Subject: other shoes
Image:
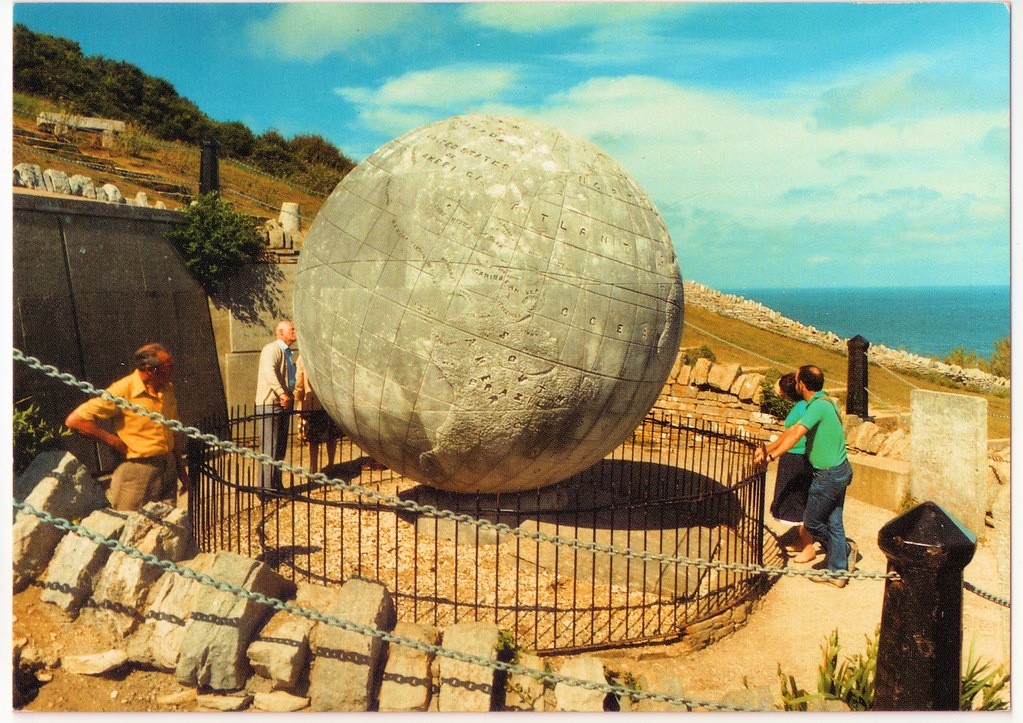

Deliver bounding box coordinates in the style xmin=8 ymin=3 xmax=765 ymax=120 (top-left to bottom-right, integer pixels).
xmin=271 ymin=482 xmax=286 ymax=490
xmin=847 ymin=541 xmax=858 ymax=574
xmin=307 ymin=477 xmax=319 ymax=489
xmin=785 ymin=539 xmax=804 ymax=552
xmin=809 ymin=574 xmax=849 ymax=589
xmin=327 ymin=465 xmax=339 ymax=476
xmin=256 ymin=493 xmax=271 ymax=502
xmin=793 ymin=548 xmax=816 ymax=563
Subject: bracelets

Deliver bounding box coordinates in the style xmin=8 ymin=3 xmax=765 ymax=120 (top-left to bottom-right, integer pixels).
xmin=762 ymin=446 xmax=768 ymax=454
xmin=769 ymin=454 xmax=774 ymax=460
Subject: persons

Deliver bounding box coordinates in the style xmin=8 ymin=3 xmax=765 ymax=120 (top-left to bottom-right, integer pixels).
xmin=256 ymin=321 xmax=297 ymax=498
xmin=294 ymin=354 xmax=338 ymax=474
xmin=754 ymin=366 xmax=857 ymax=586
xmin=64 ymin=343 xmax=191 ymax=511
